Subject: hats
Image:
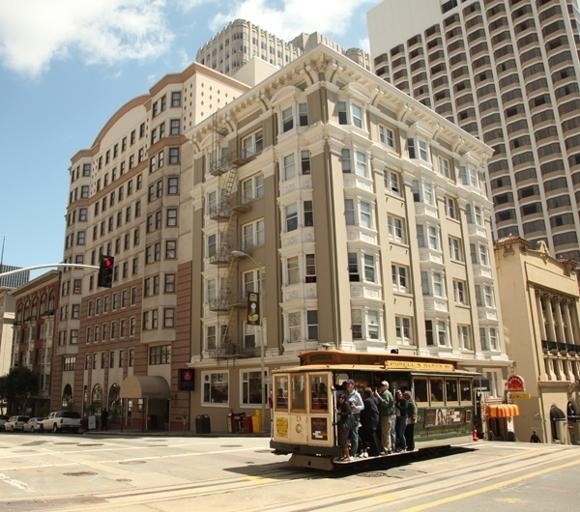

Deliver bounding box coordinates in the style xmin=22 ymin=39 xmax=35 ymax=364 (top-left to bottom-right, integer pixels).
xmin=344 ymin=379 xmax=354 ymax=384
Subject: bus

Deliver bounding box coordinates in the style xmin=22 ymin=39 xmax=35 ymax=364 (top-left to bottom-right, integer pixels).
xmin=269 ymin=348 xmax=481 ymax=470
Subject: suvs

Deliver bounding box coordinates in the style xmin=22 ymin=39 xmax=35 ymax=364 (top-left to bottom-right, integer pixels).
xmin=41 ymin=410 xmax=83 ymax=434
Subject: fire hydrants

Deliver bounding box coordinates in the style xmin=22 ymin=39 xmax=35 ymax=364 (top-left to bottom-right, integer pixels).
xmin=472 ymin=426 xmax=479 ymax=440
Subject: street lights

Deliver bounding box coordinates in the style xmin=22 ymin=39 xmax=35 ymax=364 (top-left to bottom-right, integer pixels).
xmin=229 ymin=249 xmax=267 ymax=434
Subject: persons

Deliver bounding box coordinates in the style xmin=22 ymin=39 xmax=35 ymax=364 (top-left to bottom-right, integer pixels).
xmin=101 ymin=407 xmax=109 ymax=431
xmin=528 ymin=430 xmax=542 ymax=443
xmin=276 ymin=378 xmax=417 ymax=462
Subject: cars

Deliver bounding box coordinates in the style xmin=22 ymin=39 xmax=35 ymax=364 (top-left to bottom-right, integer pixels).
xmin=0 ymin=414 xmax=44 ymax=432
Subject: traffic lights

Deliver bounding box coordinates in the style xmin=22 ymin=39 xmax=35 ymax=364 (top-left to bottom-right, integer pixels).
xmin=96 ymin=254 xmax=115 ymax=288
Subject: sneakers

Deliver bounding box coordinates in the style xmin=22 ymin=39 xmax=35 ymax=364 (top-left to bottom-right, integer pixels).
xmin=340 ymin=448 xmax=415 ymax=462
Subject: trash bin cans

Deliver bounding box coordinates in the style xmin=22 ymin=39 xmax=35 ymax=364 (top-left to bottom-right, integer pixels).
xmin=196 ymin=415 xmax=210 ymax=433
